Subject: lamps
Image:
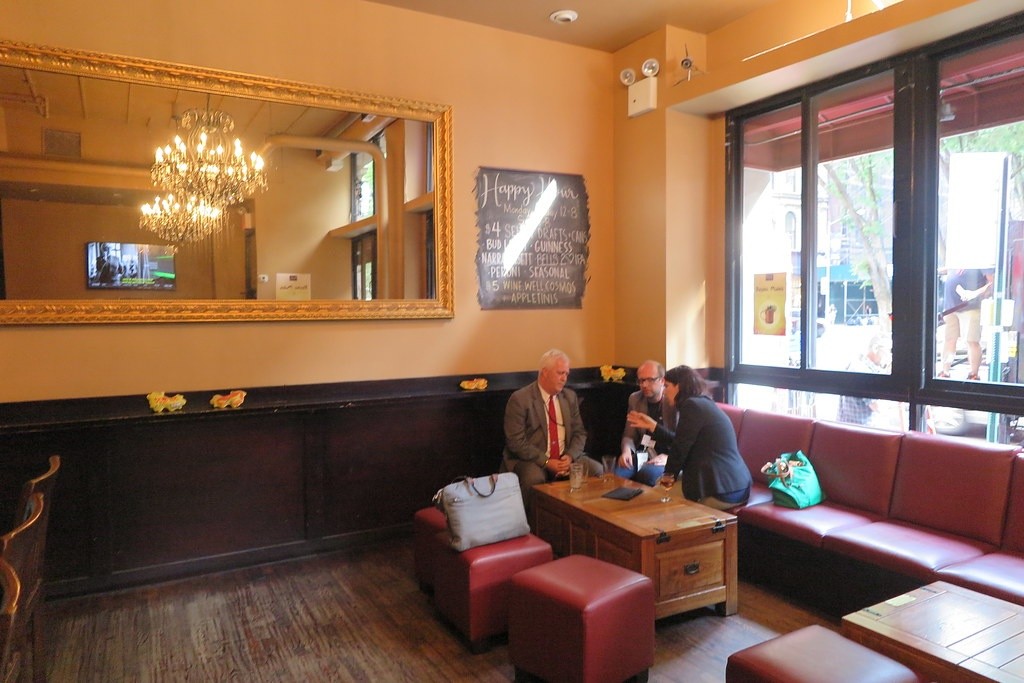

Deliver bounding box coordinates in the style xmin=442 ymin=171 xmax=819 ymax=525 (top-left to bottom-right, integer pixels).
xmin=136 ymin=93 xmax=270 ymax=244
xmin=619 ymin=58 xmax=660 ymax=117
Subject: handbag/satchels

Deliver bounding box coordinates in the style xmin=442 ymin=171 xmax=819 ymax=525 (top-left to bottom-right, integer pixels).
xmin=432 ymin=472 xmax=530 ymax=552
xmin=760 ymin=449 xmax=823 ymax=509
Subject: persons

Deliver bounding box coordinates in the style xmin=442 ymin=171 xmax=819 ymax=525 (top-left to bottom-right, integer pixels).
xmin=499 ymin=349 xmax=604 ymax=511
xmin=627 ymin=365 xmax=753 ymax=511
xmin=613 ymin=359 xmax=680 ymax=487
xmin=95 ymin=243 xmax=137 ymax=283
xmin=938 ymin=215 xmax=994 ymax=381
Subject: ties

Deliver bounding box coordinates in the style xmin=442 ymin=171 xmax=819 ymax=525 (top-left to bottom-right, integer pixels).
xmin=548 ymin=396 xmax=561 ymax=460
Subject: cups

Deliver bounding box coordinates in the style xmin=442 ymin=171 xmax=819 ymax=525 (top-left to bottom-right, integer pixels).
xmin=568 ymin=458 xmax=589 ymax=492
xmin=602 ymin=455 xmax=616 ymax=482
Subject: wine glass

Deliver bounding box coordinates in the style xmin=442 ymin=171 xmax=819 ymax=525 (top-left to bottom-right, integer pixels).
xmin=661 ymin=472 xmax=675 ymax=502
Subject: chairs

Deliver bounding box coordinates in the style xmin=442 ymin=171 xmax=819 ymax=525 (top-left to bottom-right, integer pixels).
xmin=0 ymin=455 xmax=60 ymax=683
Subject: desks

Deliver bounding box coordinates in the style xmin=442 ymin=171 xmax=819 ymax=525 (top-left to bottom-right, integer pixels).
xmin=532 ymin=468 xmax=738 ymax=619
xmin=841 ymin=581 xmax=1024 ymax=683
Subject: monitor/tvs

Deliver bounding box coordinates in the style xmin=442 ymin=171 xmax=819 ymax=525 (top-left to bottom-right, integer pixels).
xmin=87 ymin=242 xmax=176 ymax=292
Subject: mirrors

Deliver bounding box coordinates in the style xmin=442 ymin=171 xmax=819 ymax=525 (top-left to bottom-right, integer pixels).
xmin=0 ymin=37 xmax=457 ymax=323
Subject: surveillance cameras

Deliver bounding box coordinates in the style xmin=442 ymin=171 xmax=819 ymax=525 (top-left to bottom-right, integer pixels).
xmin=681 ymin=57 xmax=693 ymax=69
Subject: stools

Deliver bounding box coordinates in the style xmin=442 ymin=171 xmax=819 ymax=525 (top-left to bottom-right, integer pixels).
xmin=507 ymin=551 xmax=657 ymax=682
xmin=413 ymin=506 xmax=455 ymax=591
xmin=726 ymin=623 xmax=918 ymax=683
xmin=430 ymin=530 xmax=552 ymax=644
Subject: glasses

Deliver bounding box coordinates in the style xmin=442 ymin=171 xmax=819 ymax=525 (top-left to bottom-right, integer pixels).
xmin=635 ymin=376 xmax=661 ymax=386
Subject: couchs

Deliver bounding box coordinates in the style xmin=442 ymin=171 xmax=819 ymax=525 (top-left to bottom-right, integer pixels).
xmin=714 ymin=400 xmax=1023 ymax=603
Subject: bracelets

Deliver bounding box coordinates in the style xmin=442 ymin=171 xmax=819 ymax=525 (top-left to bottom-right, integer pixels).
xmin=543 ymin=458 xmax=549 ymax=469
